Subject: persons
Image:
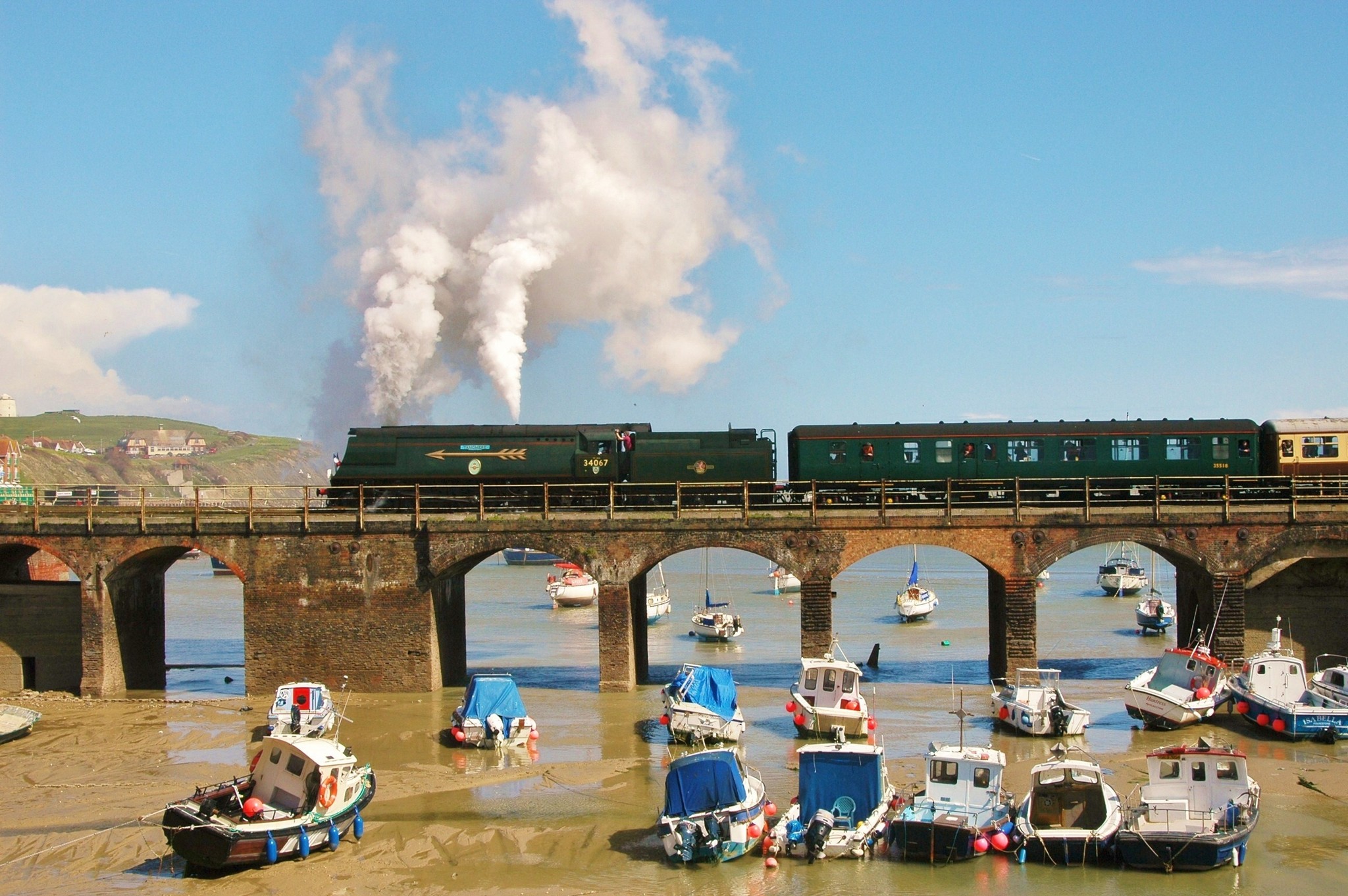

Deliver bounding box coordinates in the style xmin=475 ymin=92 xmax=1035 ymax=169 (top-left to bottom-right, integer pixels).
xmin=1238 ymin=438 xmax=1251 ymax=457
xmin=614 ymin=429 xmax=633 ymax=451
xmin=1302 ymin=438 xmax=1338 ymax=458
xmin=1282 ymin=439 xmax=1293 ymax=458
xmin=961 ymin=441 xmax=1083 ymax=461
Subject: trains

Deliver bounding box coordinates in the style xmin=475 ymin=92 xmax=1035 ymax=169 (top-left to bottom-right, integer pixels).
xmin=317 ymin=417 xmax=1348 ymax=503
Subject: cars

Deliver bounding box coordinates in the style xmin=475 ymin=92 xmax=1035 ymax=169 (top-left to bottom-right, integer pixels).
xmin=132 ymin=454 xmax=149 ymax=459
xmin=154 ymin=448 xmax=217 ymax=458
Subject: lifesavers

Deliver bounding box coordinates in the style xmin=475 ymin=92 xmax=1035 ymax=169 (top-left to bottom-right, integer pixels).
xmin=317 ymin=775 xmax=338 ymax=808
xmin=1191 ymin=676 xmax=1204 ymax=691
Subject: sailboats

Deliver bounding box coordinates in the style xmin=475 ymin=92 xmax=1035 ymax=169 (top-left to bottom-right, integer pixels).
xmin=687 ymin=548 xmax=745 ymax=640
xmin=890 ymin=544 xmax=940 ymax=622
xmin=1035 ymin=538 xmax=1176 ymax=634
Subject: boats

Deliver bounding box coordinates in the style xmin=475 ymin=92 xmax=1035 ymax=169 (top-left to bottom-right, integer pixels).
xmin=1220 ymin=614 xmax=1348 ymax=743
xmin=266 ymin=681 xmax=354 ymax=742
xmin=758 ymin=687 xmax=905 ymax=871
xmin=180 ymin=548 xmax=237 ymax=576
xmin=1004 ymin=742 xmax=1125 ymax=869
xmin=767 ymin=560 xmax=801 ymax=596
xmin=0 ymin=705 xmax=42 ymax=745
xmin=645 ymin=562 xmax=670 ymax=624
xmin=655 ymin=726 xmax=777 ymax=865
xmin=989 ymin=667 xmax=1094 ymax=738
xmin=502 ymin=547 xmax=569 ymax=566
xmin=157 ymin=730 xmax=377 ymax=874
xmin=785 ymin=631 xmax=872 ymax=740
xmin=658 ymin=662 xmax=746 ymax=745
xmin=885 ymin=687 xmax=1020 ymax=864
xmin=448 ymin=668 xmax=538 ymax=761
xmin=1106 ymin=734 xmax=1261 ymax=876
xmin=1116 ymin=576 xmax=1234 ymax=732
xmin=544 ymin=562 xmax=599 ymax=609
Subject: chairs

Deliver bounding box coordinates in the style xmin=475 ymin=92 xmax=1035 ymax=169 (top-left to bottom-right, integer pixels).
xmin=830 ymin=796 xmax=856 ymax=831
